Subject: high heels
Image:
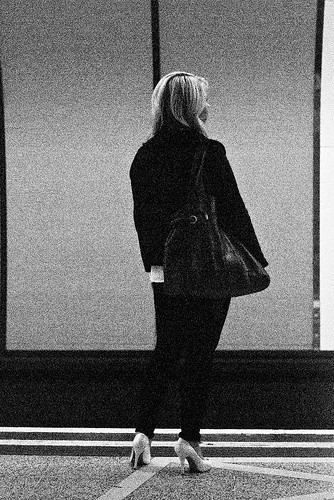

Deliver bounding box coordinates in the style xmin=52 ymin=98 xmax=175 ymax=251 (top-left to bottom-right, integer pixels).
xmin=129 ymin=433 xmax=153 ymax=469
xmin=172 ymin=438 xmax=213 ymax=474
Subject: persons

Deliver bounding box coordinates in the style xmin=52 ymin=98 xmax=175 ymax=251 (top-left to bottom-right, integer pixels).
xmin=125 ymin=71 xmax=271 ymax=471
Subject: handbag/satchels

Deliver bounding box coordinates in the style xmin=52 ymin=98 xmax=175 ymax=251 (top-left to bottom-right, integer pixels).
xmin=165 ymin=200 xmax=273 ymax=297
